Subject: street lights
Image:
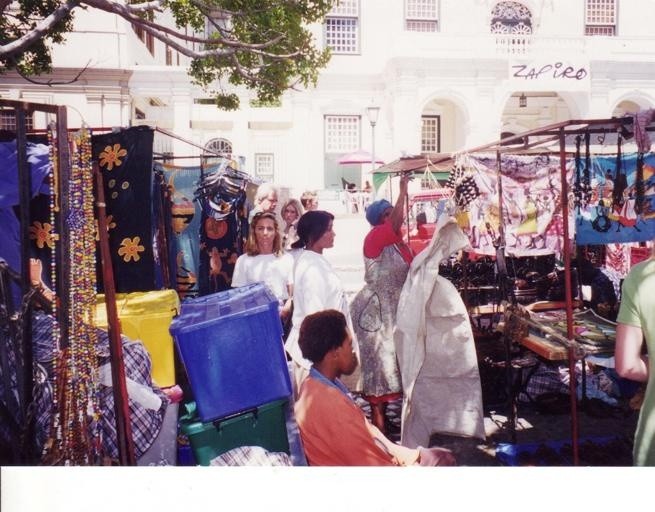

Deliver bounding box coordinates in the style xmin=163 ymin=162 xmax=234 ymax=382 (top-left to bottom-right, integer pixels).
xmin=366 ymin=106 xmax=382 ymax=173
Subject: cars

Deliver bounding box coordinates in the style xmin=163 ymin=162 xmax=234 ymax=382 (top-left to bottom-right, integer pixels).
xmin=398 ymin=187 xmax=455 ymax=259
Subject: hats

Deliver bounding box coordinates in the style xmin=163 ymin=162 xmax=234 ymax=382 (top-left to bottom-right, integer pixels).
xmin=365 ymin=199 xmax=392 ymax=227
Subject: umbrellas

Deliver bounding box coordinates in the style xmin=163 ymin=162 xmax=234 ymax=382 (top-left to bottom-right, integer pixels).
xmin=337 ymin=150 xmax=386 ymax=166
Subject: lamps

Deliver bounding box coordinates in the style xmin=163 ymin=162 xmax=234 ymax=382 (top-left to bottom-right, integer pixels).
xmin=520 ymin=93 xmax=527 ymax=107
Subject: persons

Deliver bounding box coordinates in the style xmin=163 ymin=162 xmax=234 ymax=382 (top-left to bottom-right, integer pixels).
xmin=346 ymin=171 xmax=417 ymax=434
xmin=362 ymin=180 xmax=373 ymax=213
xmin=230 ymin=210 xmax=294 ymax=359
xmin=245 ymin=182 xmax=283 ymax=246
xmin=279 ymin=197 xmax=305 ymax=251
xmin=300 ymin=190 xmax=319 ymax=214
xmin=282 ymin=209 xmax=362 ymax=396
xmin=348 ymin=182 xmax=360 ymax=213
xmin=291 ymin=308 xmax=460 ymax=466
xmin=613 ymin=239 xmax=655 ymax=466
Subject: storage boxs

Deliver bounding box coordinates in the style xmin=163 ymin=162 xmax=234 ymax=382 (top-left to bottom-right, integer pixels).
xmin=96 ymin=281 xmax=292 ymax=466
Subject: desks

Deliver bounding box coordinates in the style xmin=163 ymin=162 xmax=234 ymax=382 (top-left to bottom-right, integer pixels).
xmin=497 ymin=322 xmax=615 ymax=445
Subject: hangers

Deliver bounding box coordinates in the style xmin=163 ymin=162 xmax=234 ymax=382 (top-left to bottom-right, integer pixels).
xmin=192 ymin=156 xmax=254 ymax=203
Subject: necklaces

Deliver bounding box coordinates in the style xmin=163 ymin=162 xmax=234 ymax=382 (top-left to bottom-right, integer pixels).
xmin=43 ymin=120 xmax=106 ymax=466
xmin=573 ymin=131 xmax=646 ymax=217
